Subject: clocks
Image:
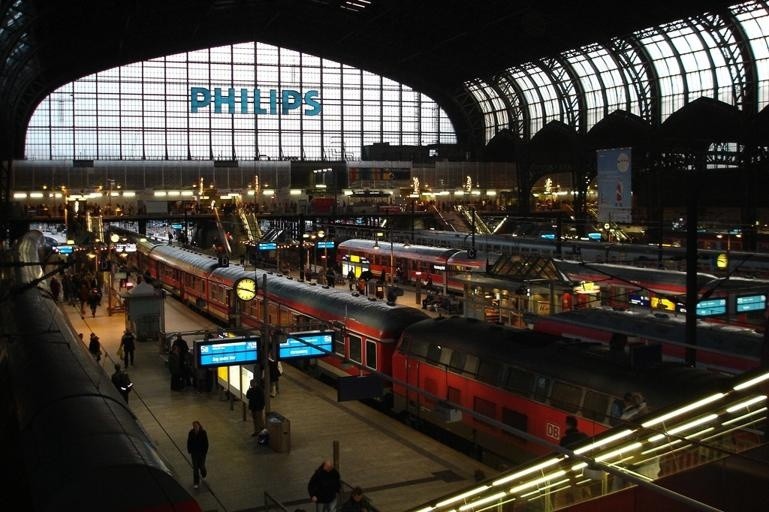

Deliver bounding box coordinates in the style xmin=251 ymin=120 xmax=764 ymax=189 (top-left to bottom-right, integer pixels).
xmin=233 ymin=278 xmax=257 ymax=301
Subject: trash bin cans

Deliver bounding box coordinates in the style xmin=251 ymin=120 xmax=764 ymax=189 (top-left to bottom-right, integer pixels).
xmin=265 ymin=412 xmax=290 ymax=453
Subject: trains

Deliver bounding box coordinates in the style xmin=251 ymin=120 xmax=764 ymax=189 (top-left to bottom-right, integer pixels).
xmin=103 ymin=222 xmax=769 ymax=511
xmin=0 ymin=230 xmax=203 ymax=512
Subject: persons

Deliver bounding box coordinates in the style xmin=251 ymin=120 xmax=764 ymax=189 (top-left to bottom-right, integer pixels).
xmin=89 ymin=332 xmax=102 ymax=364
xmin=49 ymin=216 xmax=124 ymax=319
xmin=620 ymin=391 xmax=666 ymax=486
xmin=608 ymin=389 xmax=630 ymax=491
xmin=537 ymin=198 xmax=574 ymax=211
xmin=143 ymin=199 xmax=385 ymax=295
xmin=169 ymin=333 xmax=193 ymax=390
xmin=245 ymin=376 xmax=265 ymax=438
xmin=339 ymin=487 xmax=378 ymax=512
xmin=307 ymin=461 xmax=340 ymax=512
xmin=117 ymin=328 xmax=137 ymax=368
xmin=186 ymin=420 xmax=210 ymax=491
xmin=63 ymin=199 xmax=125 ymax=217
xmin=111 ymin=364 xmax=133 ymax=405
xmin=350 ymin=196 xmax=497 ymax=213
xmin=549 ymin=415 xmax=594 ymax=507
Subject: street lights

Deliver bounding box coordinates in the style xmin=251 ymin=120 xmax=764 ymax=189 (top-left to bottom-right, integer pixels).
xmin=42 ymin=176 xmax=66 ymax=216
xmin=410 ymin=176 xmax=428 ymax=211
xmin=312 ymin=168 xmax=333 ymax=199
xmin=598 ymin=212 xmax=615 ymax=242
xmin=247 ymin=175 xmax=269 ymax=212
xmin=373 ymin=215 xmax=393 ymax=286
xmin=536 ymin=176 xmax=561 ymax=209
xmin=192 ymin=176 xmax=215 ymax=214
xmin=86 ymin=231 xmax=129 ymax=316
xmin=98 ymin=173 xmax=122 ymax=214
xmin=462 ymin=176 xmax=480 ymax=210
